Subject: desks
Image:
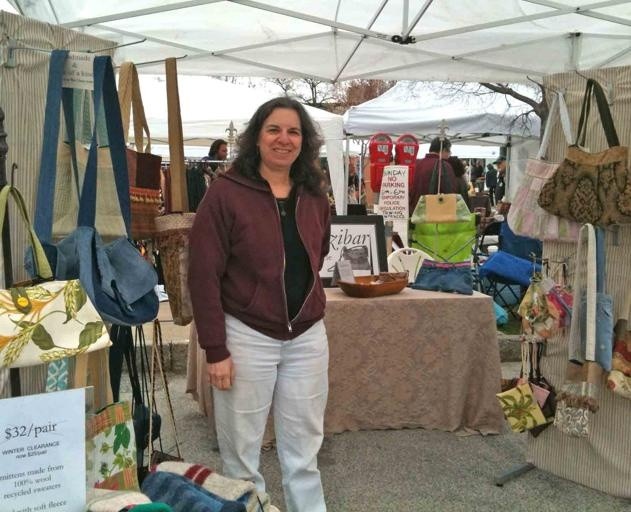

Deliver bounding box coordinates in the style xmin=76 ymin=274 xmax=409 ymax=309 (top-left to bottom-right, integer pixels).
xmin=186 ymin=285 xmax=501 ymax=447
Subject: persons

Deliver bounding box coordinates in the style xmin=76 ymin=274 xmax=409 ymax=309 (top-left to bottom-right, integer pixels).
xmin=200 ymin=140 xmax=229 ymax=171
xmin=185 ymin=97 xmax=333 ymax=511
xmin=408 ymin=134 xmax=509 ymax=217
xmin=345 ymin=158 xmax=365 ymax=201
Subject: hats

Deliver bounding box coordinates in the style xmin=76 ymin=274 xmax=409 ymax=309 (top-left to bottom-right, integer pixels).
xmin=493 ymin=156 xmax=505 ymax=164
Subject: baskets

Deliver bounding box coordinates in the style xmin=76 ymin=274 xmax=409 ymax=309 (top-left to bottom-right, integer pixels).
xmin=338 ymin=272 xmax=409 ymax=298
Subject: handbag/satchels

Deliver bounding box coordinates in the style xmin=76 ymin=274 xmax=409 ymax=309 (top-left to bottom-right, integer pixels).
xmin=85 ymin=396 xmax=184 ymax=503
xmin=496 ymin=276 xmax=631 ymax=438
xmin=411 ymin=193 xmax=476 ymax=295
xmin=470 ymin=195 xmax=492 ymax=217
xmin=0 ymin=143 xmax=196 ymax=371
xmin=506 ymin=145 xmax=631 ymax=244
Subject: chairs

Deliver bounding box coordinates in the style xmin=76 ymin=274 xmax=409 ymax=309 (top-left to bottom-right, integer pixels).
xmin=479 ymin=221 xmax=525 ymax=320
xmin=387 ymin=247 xmax=435 ymax=284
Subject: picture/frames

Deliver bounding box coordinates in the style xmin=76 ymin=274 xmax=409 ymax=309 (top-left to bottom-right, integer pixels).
xmin=318 ymin=214 xmax=388 ymax=286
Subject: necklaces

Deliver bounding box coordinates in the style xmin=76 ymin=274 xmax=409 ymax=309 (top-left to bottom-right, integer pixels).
xmin=274 ymin=193 xmax=290 ymax=217
